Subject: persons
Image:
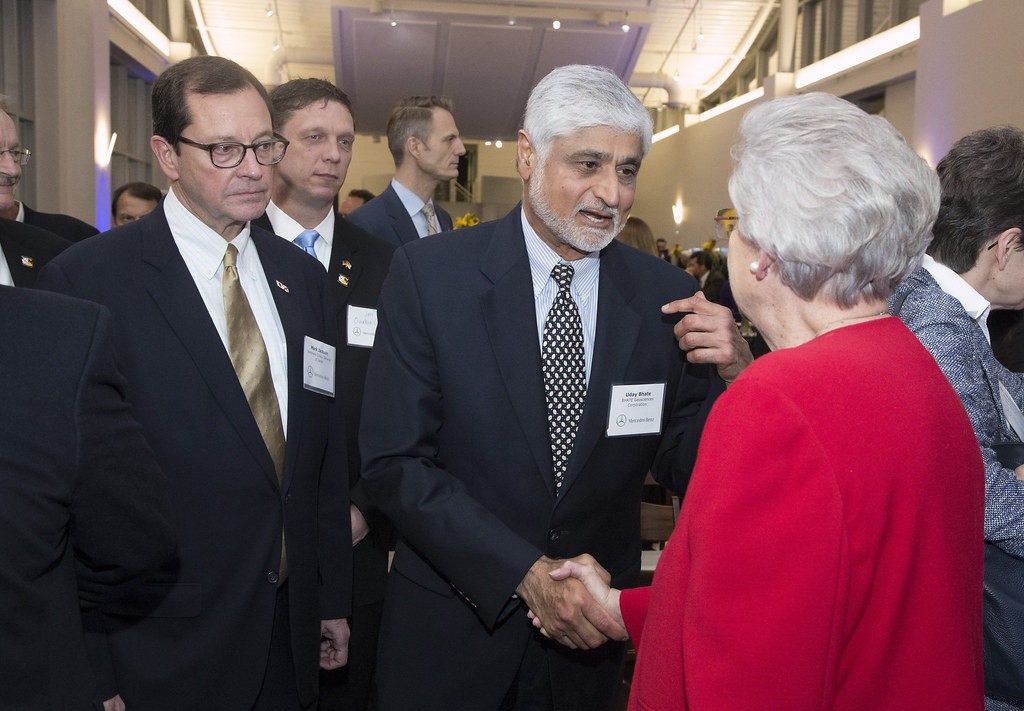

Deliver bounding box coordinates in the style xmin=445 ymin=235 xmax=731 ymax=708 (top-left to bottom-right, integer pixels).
xmin=346 ymin=95 xmax=467 ymax=247
xmin=358 ymin=64 xmax=755 ymax=711
xmin=0 ymin=216 xmax=164 ymax=711
xmin=111 ymin=181 xmax=162 ymax=228
xmin=686 ymin=250 xmax=725 ymax=303
xmin=887 ymin=128 xmax=1024 ymax=561
xmin=527 ymin=90 xmax=986 ymax=711
xmin=0 ymin=92 xmax=101 ymax=243
xmin=37 ymin=57 xmax=353 ymax=711
xmin=656 ymin=238 xmax=670 ymax=261
xmin=269 ymin=77 xmax=398 ymax=711
xmin=341 ymin=189 xmax=375 ymax=217
xmin=614 ymin=217 xmax=659 ymax=257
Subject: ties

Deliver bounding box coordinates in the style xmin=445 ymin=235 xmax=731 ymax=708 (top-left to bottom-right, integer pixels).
xmin=541 ymin=263 xmax=586 ymax=497
xmin=422 ymin=203 xmax=438 ymax=235
xmin=294 ymin=230 xmax=320 ymax=258
xmin=223 ymin=245 xmax=290 ymax=587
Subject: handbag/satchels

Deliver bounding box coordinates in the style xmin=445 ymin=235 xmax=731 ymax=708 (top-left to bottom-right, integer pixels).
xmin=980 ymin=540 xmax=1024 ymax=706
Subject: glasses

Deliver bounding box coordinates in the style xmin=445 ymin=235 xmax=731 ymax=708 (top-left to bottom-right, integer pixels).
xmin=0 ymin=148 xmax=31 ymax=165
xmin=177 ymin=131 xmax=291 ymax=168
xmin=715 ymin=207 xmax=739 ymax=240
